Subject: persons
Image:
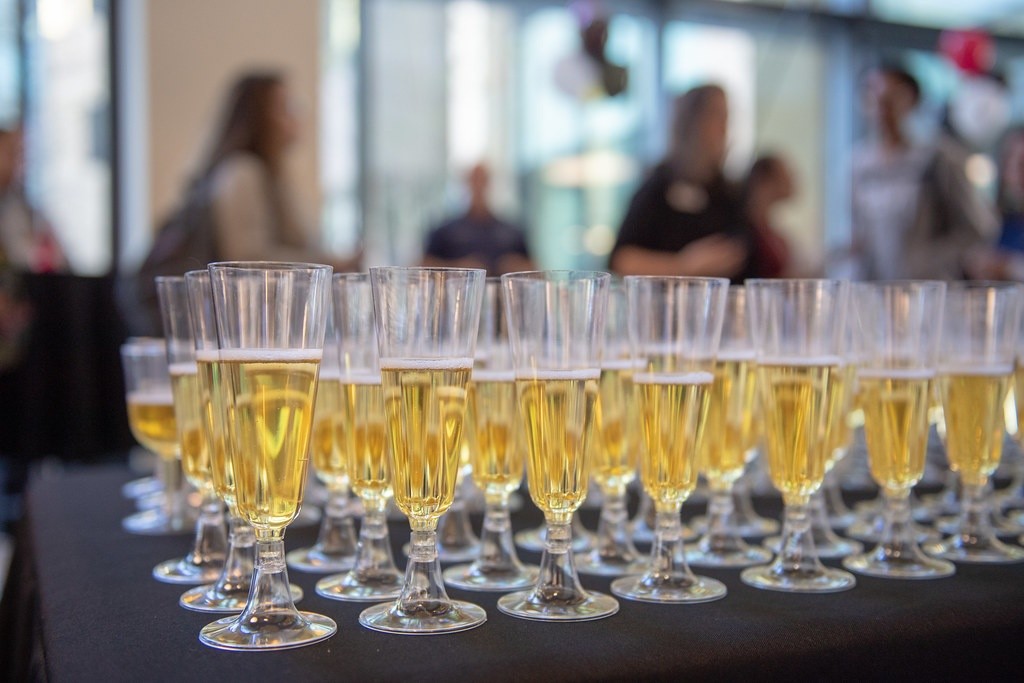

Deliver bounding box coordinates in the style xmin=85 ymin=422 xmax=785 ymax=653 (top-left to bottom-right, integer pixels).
xmin=842 ymin=61 xmax=1023 ymax=296
xmin=604 ymin=84 xmax=771 ymax=298
xmin=422 ymin=160 xmax=536 ymax=340
xmin=1 ymin=130 xmax=71 ymax=377
xmin=734 ymin=147 xmax=806 ymax=282
xmin=190 ymin=61 xmax=367 ymax=290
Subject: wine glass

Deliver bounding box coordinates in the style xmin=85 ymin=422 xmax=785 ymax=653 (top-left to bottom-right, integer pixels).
xmin=110 ymin=257 xmax=1024 ymax=653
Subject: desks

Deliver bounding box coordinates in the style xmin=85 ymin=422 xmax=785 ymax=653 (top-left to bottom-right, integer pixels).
xmin=28 ymin=414 xmax=1024 ymax=683
